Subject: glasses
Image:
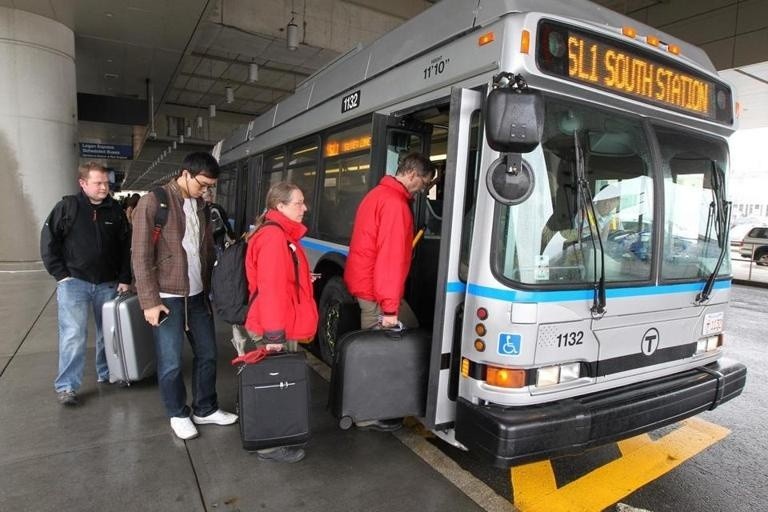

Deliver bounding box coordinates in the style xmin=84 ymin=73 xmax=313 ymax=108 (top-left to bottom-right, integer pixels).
xmin=419 ymin=176 xmax=427 ymax=188
xmin=193 ymin=177 xmax=216 ymax=188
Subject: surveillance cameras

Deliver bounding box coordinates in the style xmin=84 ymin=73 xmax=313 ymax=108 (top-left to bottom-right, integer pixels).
xmin=148 ymin=132 xmax=157 ymax=141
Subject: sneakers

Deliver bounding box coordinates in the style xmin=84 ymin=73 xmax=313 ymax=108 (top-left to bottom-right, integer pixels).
xmin=170 ymin=416 xmax=199 ymax=440
xmin=59 ymin=390 xmax=79 ymax=405
xmin=193 ymin=408 xmax=239 ymax=426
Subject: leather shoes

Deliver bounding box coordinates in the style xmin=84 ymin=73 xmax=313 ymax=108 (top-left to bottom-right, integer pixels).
xmin=356 ymin=419 xmax=404 ymax=432
xmin=256 ymin=448 xmax=304 ymax=465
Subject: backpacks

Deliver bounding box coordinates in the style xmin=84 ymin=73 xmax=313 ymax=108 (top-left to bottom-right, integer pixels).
xmin=210 ymin=222 xmax=297 ymax=324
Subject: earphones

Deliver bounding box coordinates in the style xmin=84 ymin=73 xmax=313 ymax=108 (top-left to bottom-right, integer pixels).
xmin=185 ymin=173 xmax=187 ymax=178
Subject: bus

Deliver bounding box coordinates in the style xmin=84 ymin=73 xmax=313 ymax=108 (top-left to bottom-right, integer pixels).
xmin=205 ymin=1 xmax=748 ymax=477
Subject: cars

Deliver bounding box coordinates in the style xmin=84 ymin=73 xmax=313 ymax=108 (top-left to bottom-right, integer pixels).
xmin=583 ymin=214 xmax=767 ymax=270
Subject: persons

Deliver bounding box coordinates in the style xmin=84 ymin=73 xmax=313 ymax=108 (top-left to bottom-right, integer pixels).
xmin=115 ymin=189 xmax=237 ymax=303
xmin=573 ymin=185 xmax=624 ymax=242
xmin=342 ymin=151 xmax=435 ymax=432
xmin=244 ymin=180 xmax=319 ymax=464
xmin=40 ymin=159 xmax=130 ymax=406
xmin=131 ymin=151 xmax=238 ymax=439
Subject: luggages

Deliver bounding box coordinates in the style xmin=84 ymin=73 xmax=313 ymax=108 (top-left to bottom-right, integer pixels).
xmin=240 ymin=348 xmax=311 ymax=449
xmin=100 ymin=291 xmax=157 ymax=388
xmin=330 ymin=315 xmax=429 ymax=431
